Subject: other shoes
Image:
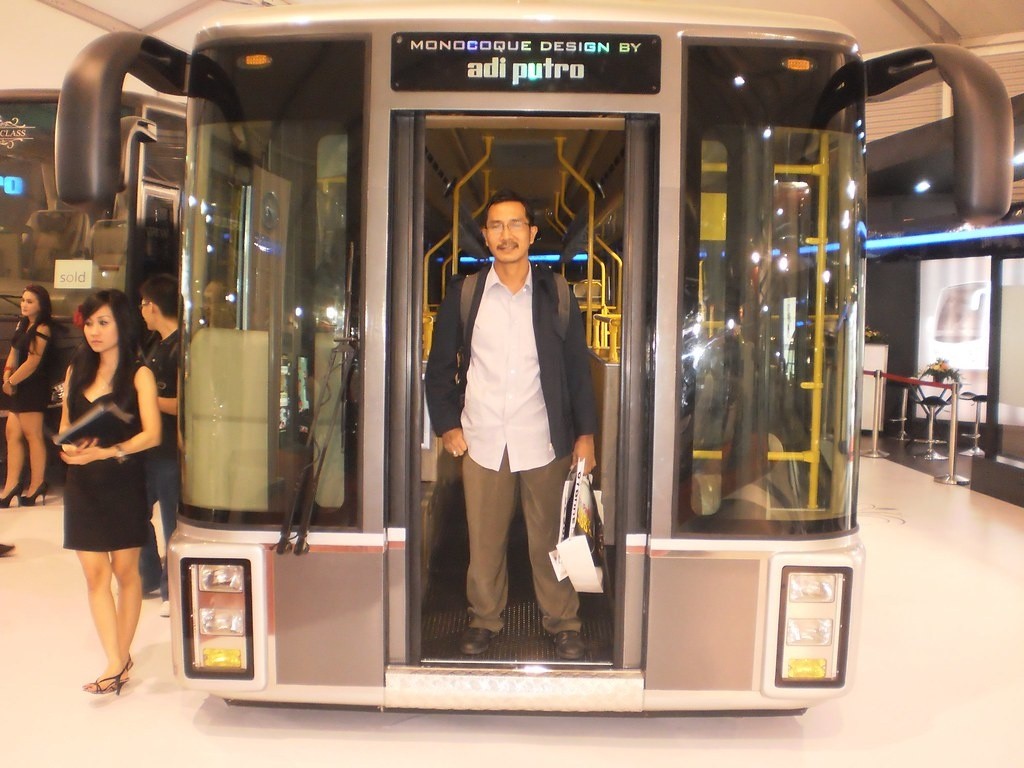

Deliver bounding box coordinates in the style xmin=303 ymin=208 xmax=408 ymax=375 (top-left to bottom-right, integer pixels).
xmin=0 ymin=544 xmax=14 ymax=556
xmin=161 ymin=600 xmax=169 ymax=617
xmin=114 ymin=580 xmax=160 ymax=595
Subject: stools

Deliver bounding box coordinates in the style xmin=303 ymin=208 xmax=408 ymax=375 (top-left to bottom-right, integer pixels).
xmin=958 ymin=392 xmax=987 ymax=457
xmin=910 ymin=394 xmax=953 ymax=461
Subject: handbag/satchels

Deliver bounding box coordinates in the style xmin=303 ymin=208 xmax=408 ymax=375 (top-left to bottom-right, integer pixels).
xmin=547 ymin=458 xmax=606 ymax=594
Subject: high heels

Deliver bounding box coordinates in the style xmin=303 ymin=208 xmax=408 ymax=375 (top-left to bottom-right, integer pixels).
xmin=83 ymin=668 xmax=129 ymax=695
xmin=21 ymin=480 xmax=47 ymax=506
xmin=125 ymin=656 xmax=133 ymax=671
xmin=0 ymin=481 xmax=24 ymax=507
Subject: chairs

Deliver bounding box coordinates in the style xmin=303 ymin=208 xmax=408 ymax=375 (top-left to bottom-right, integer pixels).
xmin=573 ymin=279 xmax=601 ymax=300
xmin=23 ymin=210 xmax=84 ymax=270
xmin=87 ymin=218 xmax=128 ymax=267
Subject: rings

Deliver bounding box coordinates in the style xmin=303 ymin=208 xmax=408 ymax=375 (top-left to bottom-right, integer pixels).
xmin=453 ymin=452 xmax=457 ymax=457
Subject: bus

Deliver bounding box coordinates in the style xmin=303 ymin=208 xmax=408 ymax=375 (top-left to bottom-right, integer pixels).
xmin=925 ymin=282 xmax=992 ymax=377
xmin=0 ymin=0 xmax=1012 ymax=718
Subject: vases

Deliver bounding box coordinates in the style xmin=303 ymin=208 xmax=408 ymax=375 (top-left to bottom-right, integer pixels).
xmin=933 ymin=376 xmax=945 ymax=383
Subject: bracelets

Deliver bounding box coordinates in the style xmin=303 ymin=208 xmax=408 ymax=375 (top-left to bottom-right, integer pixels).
xmin=115 ymin=445 xmax=127 ymax=458
xmin=3 ymin=367 xmax=14 ymax=372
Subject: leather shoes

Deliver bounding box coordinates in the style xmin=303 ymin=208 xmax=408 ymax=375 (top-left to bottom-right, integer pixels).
xmin=459 ymin=628 xmax=495 ymax=655
xmin=552 ymin=631 xmax=586 ymax=659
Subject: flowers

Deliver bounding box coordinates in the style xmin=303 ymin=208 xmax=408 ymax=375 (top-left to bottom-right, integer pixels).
xmin=914 ymin=358 xmax=962 ymax=383
xmin=864 ymin=323 xmax=889 ymax=345
xmin=72 ymin=305 xmax=85 ymax=330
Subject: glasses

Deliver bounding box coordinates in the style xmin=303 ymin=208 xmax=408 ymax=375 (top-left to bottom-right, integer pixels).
xmin=138 ymin=303 xmax=149 ymax=310
xmin=486 ymin=221 xmax=529 ymax=232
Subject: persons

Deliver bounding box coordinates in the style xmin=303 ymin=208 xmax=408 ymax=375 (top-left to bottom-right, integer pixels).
xmin=0 ymin=284 xmax=54 ymax=507
xmin=59 ymin=289 xmax=161 ymax=695
xmin=134 ymin=273 xmax=184 ymax=617
xmin=422 ymin=190 xmax=596 ymax=658
